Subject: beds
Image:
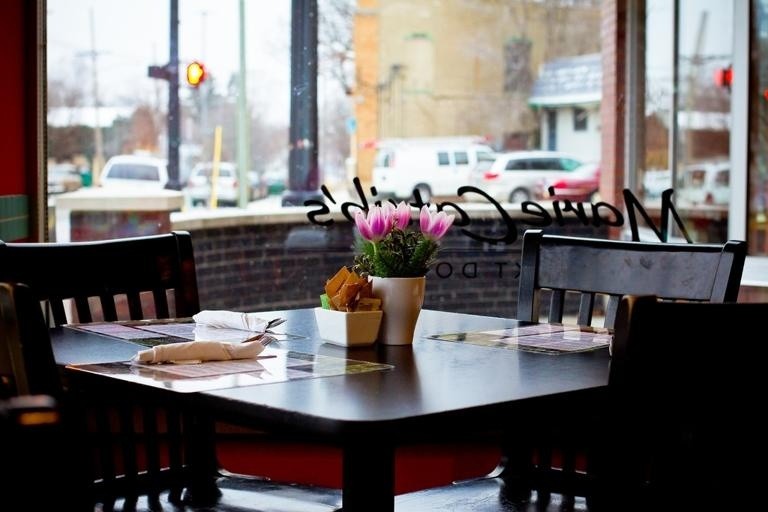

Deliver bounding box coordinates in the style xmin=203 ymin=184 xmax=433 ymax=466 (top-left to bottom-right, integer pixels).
xmin=47 ymin=307 xmax=613 ymax=512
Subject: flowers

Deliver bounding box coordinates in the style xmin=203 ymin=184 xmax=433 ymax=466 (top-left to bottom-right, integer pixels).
xmin=347 ymin=200 xmax=457 ymax=276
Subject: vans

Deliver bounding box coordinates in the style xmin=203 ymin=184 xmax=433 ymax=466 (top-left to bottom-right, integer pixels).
xmin=373 ymin=138 xmax=497 ymax=205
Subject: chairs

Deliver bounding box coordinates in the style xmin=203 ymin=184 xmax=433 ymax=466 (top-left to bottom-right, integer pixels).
xmin=394 ymin=229 xmax=768 ymax=512
xmin=2 ymin=230 xmax=344 ymax=508
xmin=612 ymin=300 xmax=768 ymax=508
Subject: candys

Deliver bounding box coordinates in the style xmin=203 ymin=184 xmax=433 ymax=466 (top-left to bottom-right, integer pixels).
xmin=320 ymin=264 xmax=381 ymax=312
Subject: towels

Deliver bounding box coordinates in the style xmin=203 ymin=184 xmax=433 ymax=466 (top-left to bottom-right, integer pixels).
xmin=191 ymin=308 xmax=269 ymax=333
xmin=132 ymin=338 xmax=264 ymax=365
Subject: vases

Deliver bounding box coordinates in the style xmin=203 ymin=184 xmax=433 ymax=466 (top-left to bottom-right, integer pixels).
xmin=367 ymin=275 xmax=427 ymax=346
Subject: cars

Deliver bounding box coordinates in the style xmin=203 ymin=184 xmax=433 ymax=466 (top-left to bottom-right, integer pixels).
xmin=46 ymin=162 xmax=83 ymax=195
xmin=95 ymin=153 xmax=270 ymax=209
xmin=461 ymin=135 xmax=730 ymax=205
